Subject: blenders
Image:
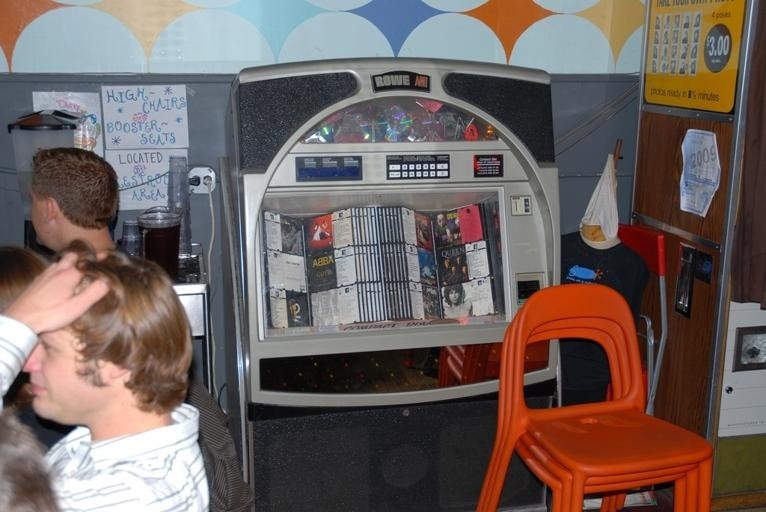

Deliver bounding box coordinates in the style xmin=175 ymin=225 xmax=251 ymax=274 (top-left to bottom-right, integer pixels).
xmin=15 ymin=109 xmax=81 ymax=268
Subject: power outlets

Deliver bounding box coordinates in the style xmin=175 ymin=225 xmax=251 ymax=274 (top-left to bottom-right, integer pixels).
xmin=188 ymin=166 xmax=216 ymax=195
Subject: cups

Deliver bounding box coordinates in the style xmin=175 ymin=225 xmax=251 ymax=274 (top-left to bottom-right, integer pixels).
xmin=121 ymin=221 xmax=141 ymax=256
xmin=169 ymin=156 xmax=193 ymax=258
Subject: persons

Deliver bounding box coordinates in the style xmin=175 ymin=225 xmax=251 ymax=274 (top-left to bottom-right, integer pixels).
xmin=0 ymin=244 xmax=48 ymax=313
xmin=417 ymin=212 xmax=473 ymax=319
xmin=0 ymin=404 xmax=64 ymax=511
xmin=0 ymin=250 xmax=212 ymax=512
xmin=29 ymin=147 xmax=124 ymax=257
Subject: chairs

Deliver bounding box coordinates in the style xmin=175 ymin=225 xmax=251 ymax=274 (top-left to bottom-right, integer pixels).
xmin=614 ymin=225 xmax=671 ymax=494
xmin=475 ymin=285 xmax=715 ymax=512
